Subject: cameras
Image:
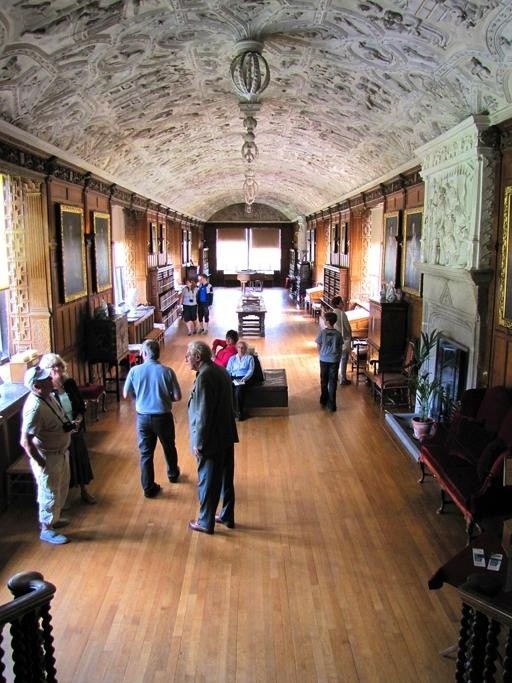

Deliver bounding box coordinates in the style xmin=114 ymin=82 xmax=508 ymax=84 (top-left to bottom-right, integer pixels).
xmin=63 ymin=421 xmax=77 ymax=432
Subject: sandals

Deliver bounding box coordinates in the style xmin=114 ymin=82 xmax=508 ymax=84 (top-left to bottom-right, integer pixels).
xmin=83 ymin=490 xmax=98 ymax=503
xmin=63 ymin=498 xmax=73 ymax=509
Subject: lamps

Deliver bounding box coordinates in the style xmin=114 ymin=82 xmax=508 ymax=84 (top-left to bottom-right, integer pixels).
xmin=229 ymin=45 xmax=272 ymax=208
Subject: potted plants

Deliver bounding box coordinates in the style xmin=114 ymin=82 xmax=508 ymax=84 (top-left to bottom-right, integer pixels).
xmin=403 ymin=325 xmax=461 ymax=441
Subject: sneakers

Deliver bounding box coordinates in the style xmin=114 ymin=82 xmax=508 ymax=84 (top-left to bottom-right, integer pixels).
xmin=54 ymin=516 xmax=71 ymax=528
xmin=321 ymin=396 xmax=336 ymax=411
xmin=188 ymin=327 xmax=208 ymax=335
xmin=340 ymin=379 xmax=352 ymax=386
xmin=41 ymin=528 xmax=68 ymax=544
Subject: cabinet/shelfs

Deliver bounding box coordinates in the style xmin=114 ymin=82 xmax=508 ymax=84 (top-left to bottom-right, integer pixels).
xmin=131 ymin=303 xmax=158 ymax=355
xmin=366 ymin=298 xmax=409 ymax=383
xmin=88 ymin=312 xmax=127 ymax=401
xmin=150 ymin=264 xmax=180 ymax=328
xmin=318 ymin=264 xmax=348 ymax=331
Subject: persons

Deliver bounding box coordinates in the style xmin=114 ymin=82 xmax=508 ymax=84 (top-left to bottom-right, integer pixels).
xmin=181 ymin=277 xmax=198 ymax=335
xmin=20 ymin=367 xmax=84 ymax=544
xmin=186 ymin=341 xmax=239 ymax=534
xmin=226 ymin=341 xmax=255 ymax=422
xmin=196 ymin=274 xmax=213 ymax=335
xmin=123 ymin=339 xmax=183 ymax=497
xmin=40 ymin=353 xmax=96 ymax=508
xmin=196 ymin=330 xmax=238 ymax=378
xmin=315 ymin=313 xmax=344 ymax=411
xmin=332 ymin=296 xmax=353 ymax=384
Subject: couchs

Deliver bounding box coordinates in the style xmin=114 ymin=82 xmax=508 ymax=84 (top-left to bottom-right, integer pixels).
xmin=419 ymin=384 xmax=512 ymax=537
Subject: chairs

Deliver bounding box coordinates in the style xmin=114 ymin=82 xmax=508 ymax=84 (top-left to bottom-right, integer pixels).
xmin=351 ymin=338 xmax=367 ymax=380
xmin=370 ymin=341 xmax=418 ymax=411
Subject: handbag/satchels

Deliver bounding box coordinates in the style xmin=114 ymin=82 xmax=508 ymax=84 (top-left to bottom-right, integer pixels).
xmin=340 ymin=310 xmax=352 ymax=353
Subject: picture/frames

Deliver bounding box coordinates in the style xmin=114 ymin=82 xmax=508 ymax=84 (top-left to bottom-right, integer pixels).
xmin=306 ymin=230 xmax=310 ymax=263
xmin=340 ymin=222 xmax=347 ymax=255
xmin=383 ymin=212 xmax=400 ymax=293
xmin=0 ymin=287 xmax=11 ymax=368
xmin=92 ymin=210 xmax=116 ymax=293
xmin=151 ymin=221 xmax=158 ymax=257
xmin=498 ymin=185 xmax=512 ymax=331
xmin=58 ymin=203 xmax=88 ymax=303
xmin=400 ymin=205 xmax=428 ymax=299
xmin=310 ymin=229 xmax=315 ymax=267
xmin=189 ymin=231 xmax=192 ymax=263
xmin=161 ymin=224 xmax=167 ymax=255
xmin=332 ymin=224 xmax=338 ymax=254
xmin=183 ymin=229 xmax=188 ymax=265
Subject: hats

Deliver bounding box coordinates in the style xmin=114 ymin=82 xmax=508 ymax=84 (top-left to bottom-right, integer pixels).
xmin=24 ymin=365 xmax=50 ymax=385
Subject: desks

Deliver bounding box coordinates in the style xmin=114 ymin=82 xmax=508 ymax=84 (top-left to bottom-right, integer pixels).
xmin=235 ymin=294 xmax=268 ymax=338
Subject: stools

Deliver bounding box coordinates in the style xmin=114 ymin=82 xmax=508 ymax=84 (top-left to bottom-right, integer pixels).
xmin=79 ymin=384 xmax=107 ymax=421
xmin=234 ymin=369 xmax=289 ymax=417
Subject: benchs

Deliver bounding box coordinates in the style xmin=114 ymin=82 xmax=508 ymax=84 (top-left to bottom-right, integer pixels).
xmin=143 ymin=330 xmax=164 ymax=355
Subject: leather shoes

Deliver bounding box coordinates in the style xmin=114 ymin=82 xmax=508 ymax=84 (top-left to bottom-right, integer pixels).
xmin=189 ymin=519 xmax=215 ymax=535
xmin=169 ymin=466 xmax=180 ymax=481
xmin=215 ymin=514 xmax=235 ymax=527
xmin=144 ymin=484 xmax=160 ymax=498
xmin=235 ymin=412 xmax=244 ymax=421
